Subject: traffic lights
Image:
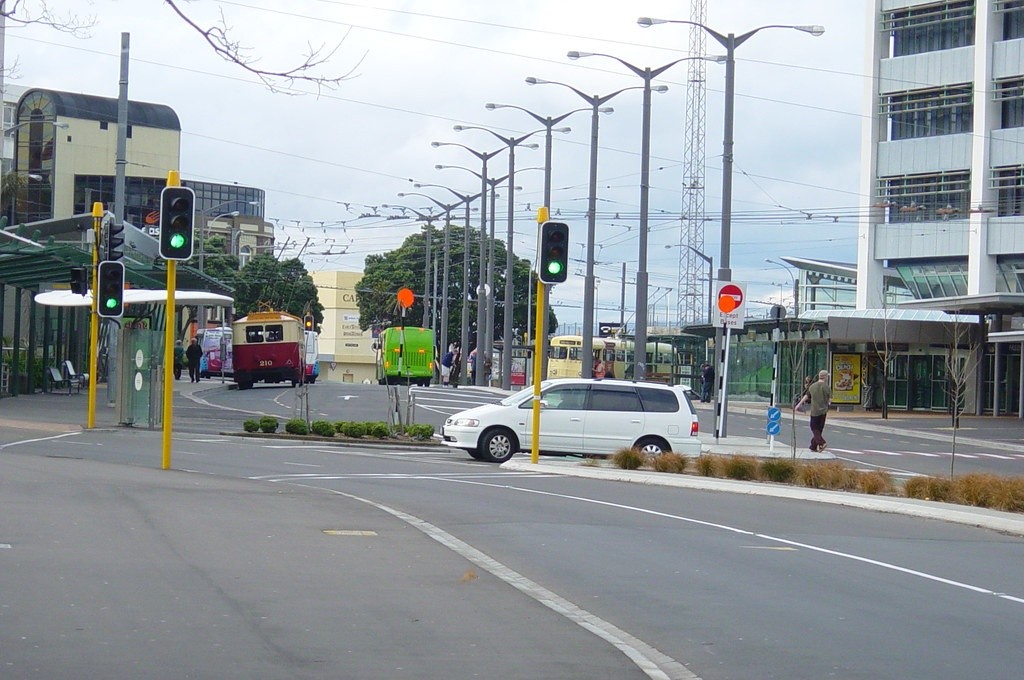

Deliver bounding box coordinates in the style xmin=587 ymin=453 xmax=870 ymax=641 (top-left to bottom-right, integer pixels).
xmin=538 ymin=221 xmax=570 ymax=284
xmin=70 ymin=267 xmax=87 ymax=295
xmin=304 ymin=316 xmax=313 ymax=331
xmin=104 ymin=220 xmax=125 ymax=260
xmin=97 ymin=261 xmax=125 ymax=317
xmin=160 ymin=186 xmax=195 ymax=261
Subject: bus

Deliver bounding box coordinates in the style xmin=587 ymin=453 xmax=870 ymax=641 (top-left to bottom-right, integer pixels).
xmin=372 ymin=327 xmax=434 ymax=387
xmin=546 ymin=336 xmax=681 ymax=383
xmin=196 ymin=299 xmax=319 ymax=391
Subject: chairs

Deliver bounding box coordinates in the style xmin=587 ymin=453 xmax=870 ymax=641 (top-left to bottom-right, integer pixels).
xmin=45 ymin=360 xmax=89 ymax=395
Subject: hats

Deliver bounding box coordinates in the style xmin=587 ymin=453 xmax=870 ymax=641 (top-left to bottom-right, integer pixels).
xmin=819 ymin=370 xmax=830 ymax=378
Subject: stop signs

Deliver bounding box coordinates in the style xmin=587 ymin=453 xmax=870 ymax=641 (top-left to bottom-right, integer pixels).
xmin=711 ymin=280 xmax=746 ymax=330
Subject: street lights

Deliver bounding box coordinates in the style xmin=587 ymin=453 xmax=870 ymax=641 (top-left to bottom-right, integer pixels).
xmin=566 ymin=50 xmax=729 ymax=380
xmin=637 ymin=16 xmax=825 ymax=434
xmin=200 ymin=200 xmax=262 ymax=272
xmin=0 ymin=120 xmax=69 ymax=179
xmin=765 ymin=260 xmax=796 ymax=299
xmin=383 ymin=77 xmax=668 ymax=392
xmin=664 ymin=245 xmax=712 ymax=324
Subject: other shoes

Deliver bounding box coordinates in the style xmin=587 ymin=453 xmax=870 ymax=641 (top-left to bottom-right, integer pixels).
xmin=819 ymin=443 xmax=827 ymax=452
xmin=809 ymin=448 xmax=817 ymax=452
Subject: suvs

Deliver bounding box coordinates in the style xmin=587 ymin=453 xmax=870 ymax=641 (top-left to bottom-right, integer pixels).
xmin=439 ymin=377 xmax=701 ymax=464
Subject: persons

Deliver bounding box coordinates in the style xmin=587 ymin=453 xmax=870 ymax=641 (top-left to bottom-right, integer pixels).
xmin=624 ymin=364 xmax=634 ymax=379
xmin=442 ymin=344 xmax=458 ymax=387
xmin=470 ymin=348 xmax=476 ymax=386
xmin=186 ymin=338 xmax=203 ymax=383
xmin=484 ymin=351 xmax=492 ymax=386
xmin=701 ymin=364 xmax=714 ymax=403
xmin=795 ymin=369 xmax=832 ymax=453
xmin=174 ymin=340 xmax=184 ymax=380
xmin=804 ymin=376 xmax=812 ymax=404
xmin=592 ymin=360 xmax=606 ymax=378
